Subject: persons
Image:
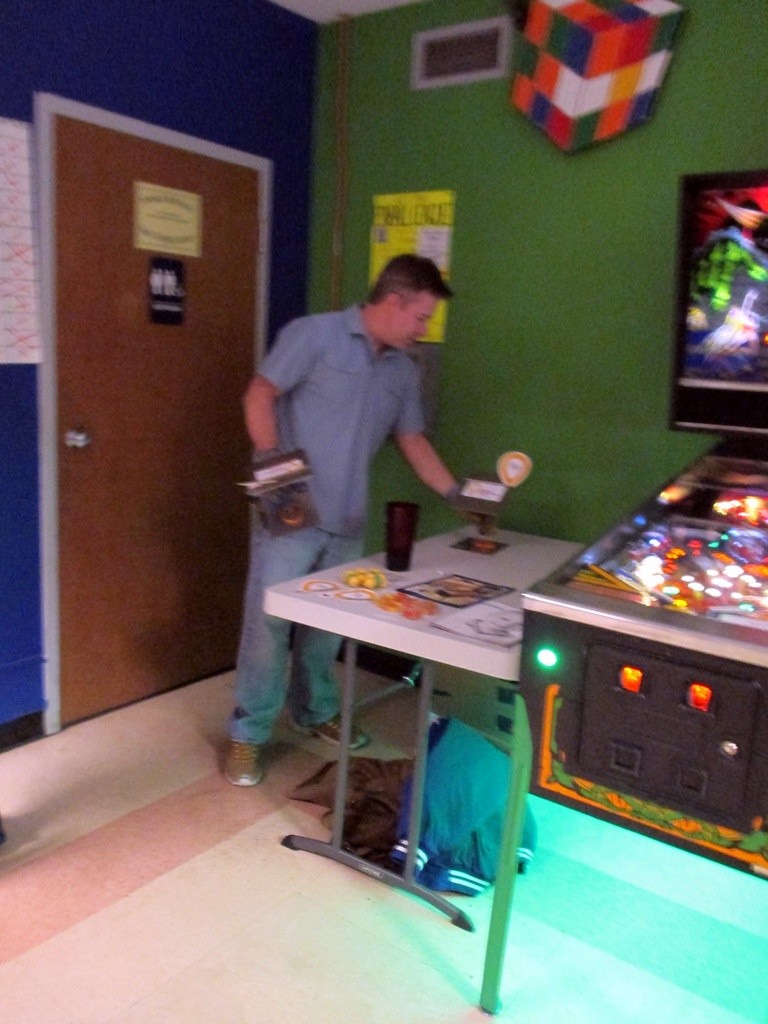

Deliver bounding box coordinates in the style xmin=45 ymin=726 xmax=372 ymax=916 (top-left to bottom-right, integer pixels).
xmin=223 ymin=253 xmax=496 ymax=787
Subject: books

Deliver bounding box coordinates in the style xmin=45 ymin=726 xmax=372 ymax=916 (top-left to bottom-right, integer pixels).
xmin=433 ymin=601 xmax=525 ymax=650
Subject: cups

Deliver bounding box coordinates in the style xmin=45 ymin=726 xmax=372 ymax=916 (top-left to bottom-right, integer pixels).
xmin=386 ymin=502 xmax=418 ymax=571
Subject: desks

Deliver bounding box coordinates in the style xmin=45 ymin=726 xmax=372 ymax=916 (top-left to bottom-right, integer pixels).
xmin=261 ymin=524 xmax=587 ymax=934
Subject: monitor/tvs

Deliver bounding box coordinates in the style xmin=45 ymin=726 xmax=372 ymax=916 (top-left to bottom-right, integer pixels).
xmin=665 ymin=168 xmax=768 ymax=438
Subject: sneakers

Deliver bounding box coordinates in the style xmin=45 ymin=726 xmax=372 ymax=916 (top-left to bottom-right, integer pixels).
xmin=294 ymin=718 xmax=367 ymax=749
xmin=225 ymin=740 xmax=262 ymax=787
